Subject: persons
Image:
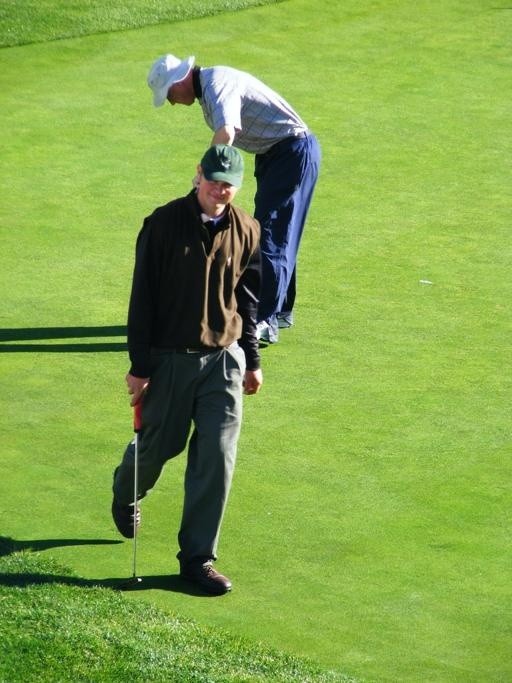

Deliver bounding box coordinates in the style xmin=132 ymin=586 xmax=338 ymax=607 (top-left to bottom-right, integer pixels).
xmin=110 ymin=142 xmax=264 ymax=596
xmin=146 ymin=51 xmax=321 ymax=346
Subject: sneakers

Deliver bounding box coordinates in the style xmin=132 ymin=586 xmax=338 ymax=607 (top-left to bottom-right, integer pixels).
xmin=255 ymin=331 xmax=269 ymax=348
xmin=276 ymin=318 xmax=291 ymax=327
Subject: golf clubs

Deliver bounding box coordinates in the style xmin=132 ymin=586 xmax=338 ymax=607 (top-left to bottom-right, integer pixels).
xmin=131 ymin=402 xmax=143 ymax=587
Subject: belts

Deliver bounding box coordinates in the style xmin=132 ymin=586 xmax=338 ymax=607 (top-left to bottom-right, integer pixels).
xmin=147 ymin=346 xmax=211 ymax=354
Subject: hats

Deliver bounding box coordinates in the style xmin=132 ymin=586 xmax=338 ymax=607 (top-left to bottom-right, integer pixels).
xmin=201 ymin=143 xmax=245 ymax=188
xmin=147 ymin=54 xmax=194 ymax=108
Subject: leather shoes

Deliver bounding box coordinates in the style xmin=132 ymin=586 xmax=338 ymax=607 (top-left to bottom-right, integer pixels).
xmin=111 ymin=467 xmax=140 ymax=538
xmin=181 ymin=562 xmax=232 ymax=594
